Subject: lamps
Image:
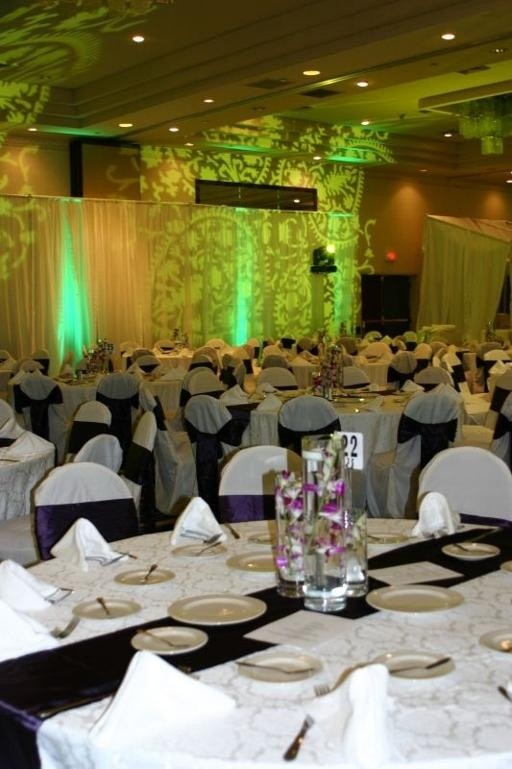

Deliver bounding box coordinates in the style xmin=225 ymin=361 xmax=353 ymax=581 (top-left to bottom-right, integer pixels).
xmin=416 ymin=78 xmax=511 ymax=156
xmin=310 ymin=245 xmax=337 ymax=273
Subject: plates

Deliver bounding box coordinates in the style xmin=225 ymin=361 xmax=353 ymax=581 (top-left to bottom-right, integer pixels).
xmin=374 ymin=650 xmax=455 ymax=682
xmin=476 ymin=625 xmax=512 ymax=654
xmin=131 ymin=628 xmax=209 ymax=655
xmin=440 ymin=543 xmax=500 ymax=562
xmin=362 ymin=580 xmax=466 ymax=614
xmin=168 ymin=594 xmax=267 ymax=624
xmin=498 ymin=559 xmax=511 ymax=575
xmin=237 ymin=649 xmax=322 ymax=683
xmin=116 ymin=530 xmax=277 ymax=584
xmin=69 ymin=599 xmax=139 ymax=622
xmin=253 ymin=389 xmax=417 ymax=415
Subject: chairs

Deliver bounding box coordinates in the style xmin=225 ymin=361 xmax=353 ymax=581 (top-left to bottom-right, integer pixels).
xmin=1 ymin=322 xmax=511 ymax=561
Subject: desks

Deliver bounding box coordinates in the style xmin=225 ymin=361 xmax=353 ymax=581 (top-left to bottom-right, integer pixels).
xmin=1 ymin=519 xmax=511 ymax=768
